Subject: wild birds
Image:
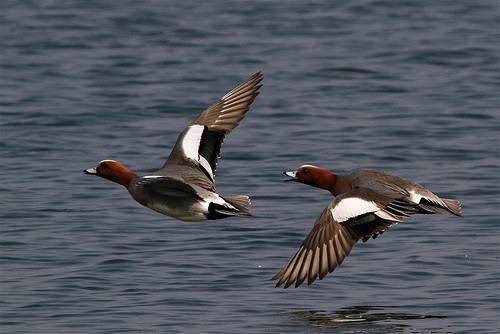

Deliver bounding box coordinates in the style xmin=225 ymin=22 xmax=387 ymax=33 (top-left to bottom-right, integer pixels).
xmin=270 ymin=165 xmax=463 ymax=289
xmin=82 ymin=70 xmax=266 ymax=222
